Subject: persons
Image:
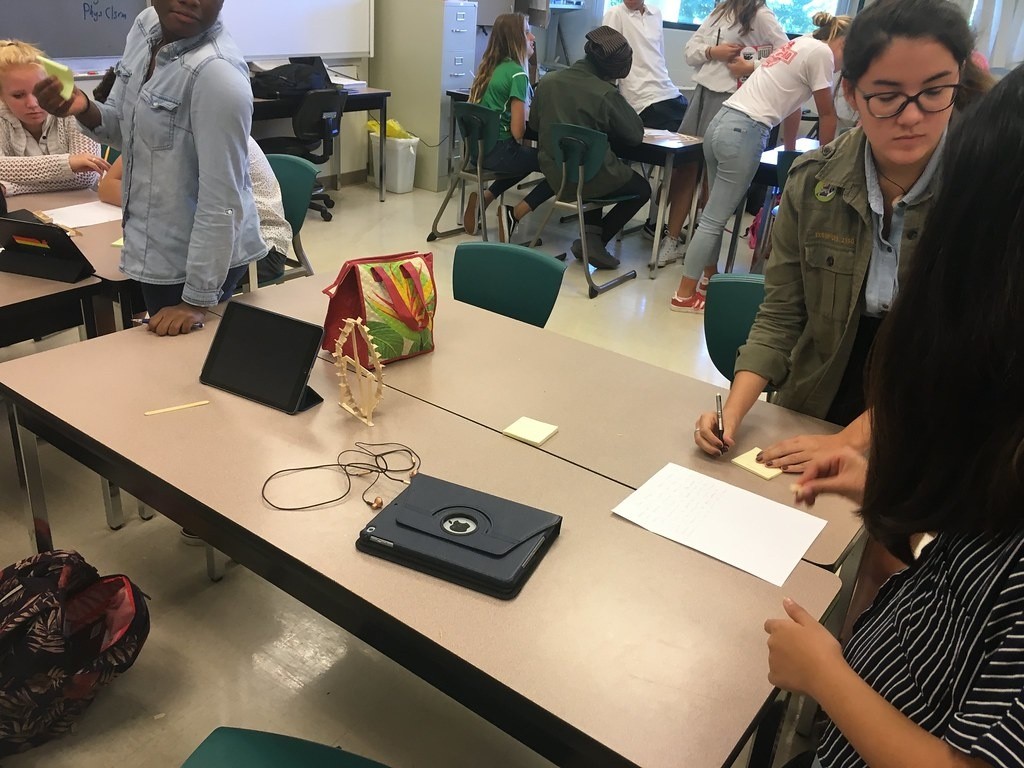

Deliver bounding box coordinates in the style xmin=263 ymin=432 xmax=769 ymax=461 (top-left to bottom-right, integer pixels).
xmin=0 ymin=37 xmax=111 ymax=197
xmin=602 ymin=0 xmax=689 ymax=134
xmin=529 ymin=26 xmax=652 ymax=270
xmin=32 ymin=0 xmax=270 ymax=545
xmin=670 ymin=11 xmax=854 ymax=314
xmin=694 ymin=0 xmax=1000 ymax=474
xmin=764 ymin=63 xmax=1024 ymax=768
xmin=93 ymin=65 xmax=293 ymax=291
xmin=463 ymin=13 xmax=557 ymax=245
xmin=647 ymin=0 xmax=802 ymax=269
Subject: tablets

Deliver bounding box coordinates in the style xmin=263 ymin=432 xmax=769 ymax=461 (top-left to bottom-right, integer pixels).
xmin=360 ymin=473 xmax=560 ymax=584
xmin=199 ymin=300 xmax=325 ymax=414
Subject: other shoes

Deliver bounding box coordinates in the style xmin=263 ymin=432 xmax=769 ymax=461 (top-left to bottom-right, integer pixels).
xmin=571 ymin=232 xmax=620 ymax=270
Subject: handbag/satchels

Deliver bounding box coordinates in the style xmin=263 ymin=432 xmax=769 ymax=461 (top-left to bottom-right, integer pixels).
xmin=249 ymin=62 xmax=327 ymax=97
xmin=321 ymin=252 xmax=438 ymax=369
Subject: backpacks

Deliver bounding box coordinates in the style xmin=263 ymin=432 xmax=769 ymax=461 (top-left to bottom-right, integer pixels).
xmin=0 ymin=548 xmax=150 ymax=758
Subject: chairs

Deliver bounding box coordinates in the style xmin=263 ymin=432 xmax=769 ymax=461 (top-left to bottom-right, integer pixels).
xmin=530 ymin=123 xmax=640 ymax=299
xmin=179 ymin=726 xmax=391 ymax=768
xmin=772 ymin=151 xmax=804 ymax=216
xmin=704 ymin=273 xmax=790 ymax=403
xmin=256 ymin=89 xmax=349 ymax=222
xmin=428 ymin=101 xmax=542 ymax=247
xmin=232 ymin=154 xmax=321 ymax=294
xmin=452 ymin=241 xmax=568 ymax=329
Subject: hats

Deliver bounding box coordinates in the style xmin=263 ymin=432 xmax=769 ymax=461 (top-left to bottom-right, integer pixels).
xmin=585 ymin=24 xmax=633 ymax=79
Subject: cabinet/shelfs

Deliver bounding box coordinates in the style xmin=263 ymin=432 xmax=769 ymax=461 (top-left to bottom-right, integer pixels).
xmin=369 ymin=0 xmax=478 ymax=193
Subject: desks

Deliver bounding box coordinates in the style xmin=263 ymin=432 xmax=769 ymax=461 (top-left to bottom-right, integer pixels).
xmin=251 ymin=85 xmax=391 ymax=203
xmin=0 ymin=272 xmax=870 ymax=768
xmin=0 ymin=188 xmax=257 ymax=554
xmin=446 ymin=89 xmax=706 ymax=280
xmin=724 ymin=137 xmax=820 ymax=273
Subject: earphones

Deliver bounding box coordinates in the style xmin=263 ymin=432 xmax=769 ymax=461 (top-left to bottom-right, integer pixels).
xmin=410 ymin=470 xmax=419 ymax=479
xmin=365 ymin=497 xmax=383 ymax=510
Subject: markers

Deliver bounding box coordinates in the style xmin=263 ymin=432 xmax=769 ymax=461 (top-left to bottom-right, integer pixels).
xmin=87 ymin=71 xmax=100 ymax=74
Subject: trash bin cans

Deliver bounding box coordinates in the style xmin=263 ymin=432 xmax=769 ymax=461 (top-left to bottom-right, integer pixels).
xmin=369 ymin=133 xmax=420 ymax=193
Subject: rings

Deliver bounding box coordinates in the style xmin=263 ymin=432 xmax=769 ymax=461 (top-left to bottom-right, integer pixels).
xmin=694 ymin=428 xmax=700 ymax=433
xmin=92 ymin=158 xmax=96 ymax=162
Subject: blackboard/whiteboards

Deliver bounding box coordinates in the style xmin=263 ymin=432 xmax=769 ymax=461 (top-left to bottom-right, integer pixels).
xmin=0 ymin=0 xmax=374 ymax=61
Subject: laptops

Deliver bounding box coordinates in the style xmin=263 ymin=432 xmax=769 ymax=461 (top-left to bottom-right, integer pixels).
xmin=290 ymin=55 xmax=360 ymax=94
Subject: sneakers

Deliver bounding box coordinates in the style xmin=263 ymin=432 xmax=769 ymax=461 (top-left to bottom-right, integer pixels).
xmin=463 ymin=190 xmax=482 ymax=235
xmin=641 ymin=220 xmax=685 ymax=246
xmin=655 ymin=230 xmax=680 ymax=268
xmin=698 ymin=276 xmax=712 ymax=297
xmin=496 ymin=205 xmax=518 ymax=244
xmin=670 ymin=287 xmax=708 ymax=314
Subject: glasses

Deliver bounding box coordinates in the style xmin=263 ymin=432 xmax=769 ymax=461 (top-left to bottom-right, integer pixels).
xmin=846 ymin=73 xmax=964 ymax=118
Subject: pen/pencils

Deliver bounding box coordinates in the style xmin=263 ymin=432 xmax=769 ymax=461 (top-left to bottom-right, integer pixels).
xmin=131 ymin=318 xmax=204 ymax=328
xmin=715 ymin=394 xmax=727 ymax=453
xmin=716 ymin=26 xmax=721 ymax=46
xmin=97 ymin=145 xmax=110 ymax=187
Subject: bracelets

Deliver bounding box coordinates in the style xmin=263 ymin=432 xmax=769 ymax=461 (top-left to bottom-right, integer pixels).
xmin=78 ymin=89 xmax=90 ymax=117
